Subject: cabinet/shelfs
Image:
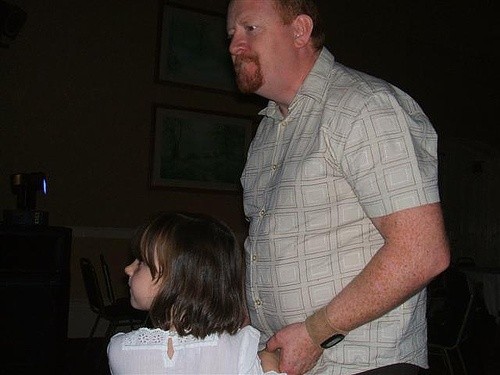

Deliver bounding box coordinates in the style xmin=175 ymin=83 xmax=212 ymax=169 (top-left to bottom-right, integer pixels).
xmin=0 ymin=225 xmax=72 ymax=374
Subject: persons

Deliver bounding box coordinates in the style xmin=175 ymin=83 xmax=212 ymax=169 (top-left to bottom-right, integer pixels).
xmin=103 ymin=207 xmax=282 ymax=375
xmin=223 ymin=0 xmax=453 ymax=375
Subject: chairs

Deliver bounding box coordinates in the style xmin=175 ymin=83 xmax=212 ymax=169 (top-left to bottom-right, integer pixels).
xmin=80 ymin=254 xmax=154 ymax=374
xmin=426 ymin=265 xmax=482 ymax=375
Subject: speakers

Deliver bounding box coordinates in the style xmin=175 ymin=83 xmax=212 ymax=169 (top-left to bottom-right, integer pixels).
xmin=0 ymin=0 xmax=27 ymax=48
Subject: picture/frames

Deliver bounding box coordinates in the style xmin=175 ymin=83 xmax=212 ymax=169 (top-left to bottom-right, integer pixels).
xmin=149 ymin=102 xmax=257 ymax=196
xmin=154 ymin=2 xmax=266 ymax=102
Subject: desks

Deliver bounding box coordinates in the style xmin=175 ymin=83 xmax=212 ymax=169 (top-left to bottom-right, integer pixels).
xmin=481 ymin=269 xmax=500 ymax=328
